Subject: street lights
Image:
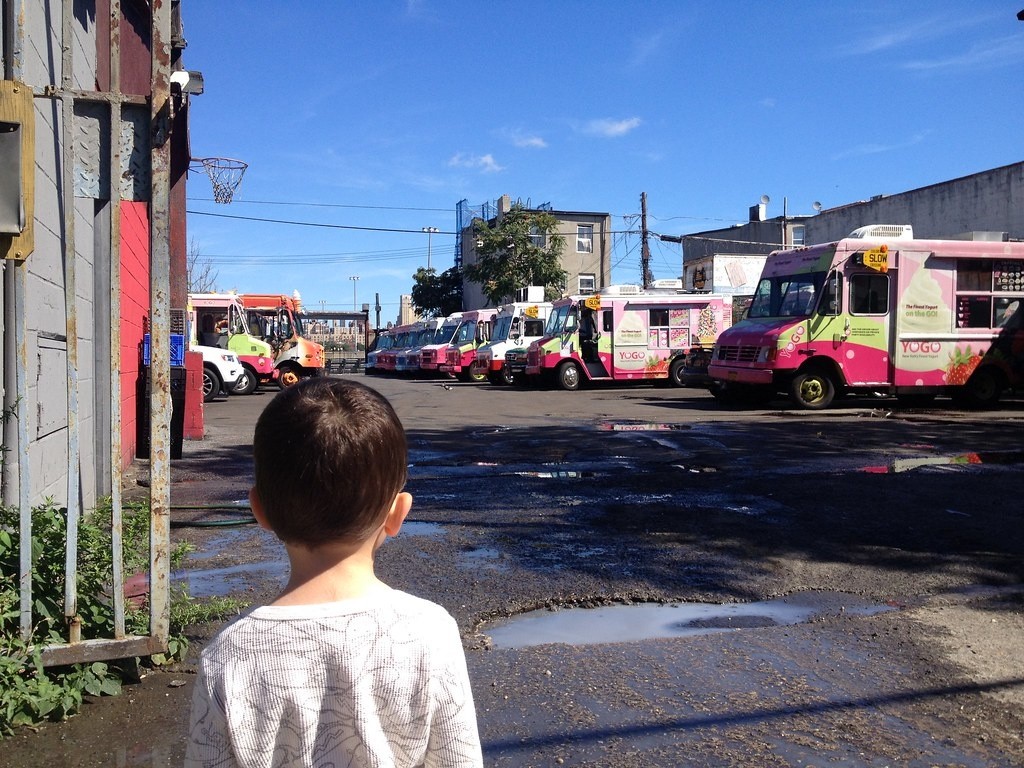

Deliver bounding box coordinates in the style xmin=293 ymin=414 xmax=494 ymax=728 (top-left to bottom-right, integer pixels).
xmin=319 ymin=301 xmax=327 ymax=346
xmin=349 ymin=277 xmax=360 ymax=351
xmin=422 ymin=227 xmax=439 ymax=269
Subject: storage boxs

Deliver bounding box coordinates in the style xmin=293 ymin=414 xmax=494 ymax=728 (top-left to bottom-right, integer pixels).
xmin=136 ymin=308 xmax=188 ymax=460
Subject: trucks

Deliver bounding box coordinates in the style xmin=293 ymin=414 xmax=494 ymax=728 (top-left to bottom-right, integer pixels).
xmin=474 ymin=286 xmax=573 ymax=387
xmin=526 ymin=279 xmax=732 ymax=391
xmin=366 ymin=317 xmax=447 ymax=379
xmin=680 ymin=285 xmax=815 ymax=399
xmin=187 ymin=294 xmax=274 ymax=396
xmin=707 ymin=224 xmax=1024 ymax=411
xmin=215 ymin=294 xmax=324 ymax=390
xmin=420 ymin=312 xmax=463 ymax=380
xmin=440 ymin=308 xmax=502 ymax=382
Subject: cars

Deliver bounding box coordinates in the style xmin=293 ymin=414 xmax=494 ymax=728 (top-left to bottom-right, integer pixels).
xmin=189 ymin=345 xmax=244 ymax=403
xmin=504 ymin=348 xmax=528 ymax=373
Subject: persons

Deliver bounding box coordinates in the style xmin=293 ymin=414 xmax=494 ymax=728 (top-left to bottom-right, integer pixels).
xmin=184 ymin=377 xmax=483 ymax=768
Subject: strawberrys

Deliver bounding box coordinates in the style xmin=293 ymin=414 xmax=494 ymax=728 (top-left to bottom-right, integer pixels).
xmin=644 ymin=354 xmax=672 ymax=379
xmin=946 ymin=345 xmax=981 ymax=385
